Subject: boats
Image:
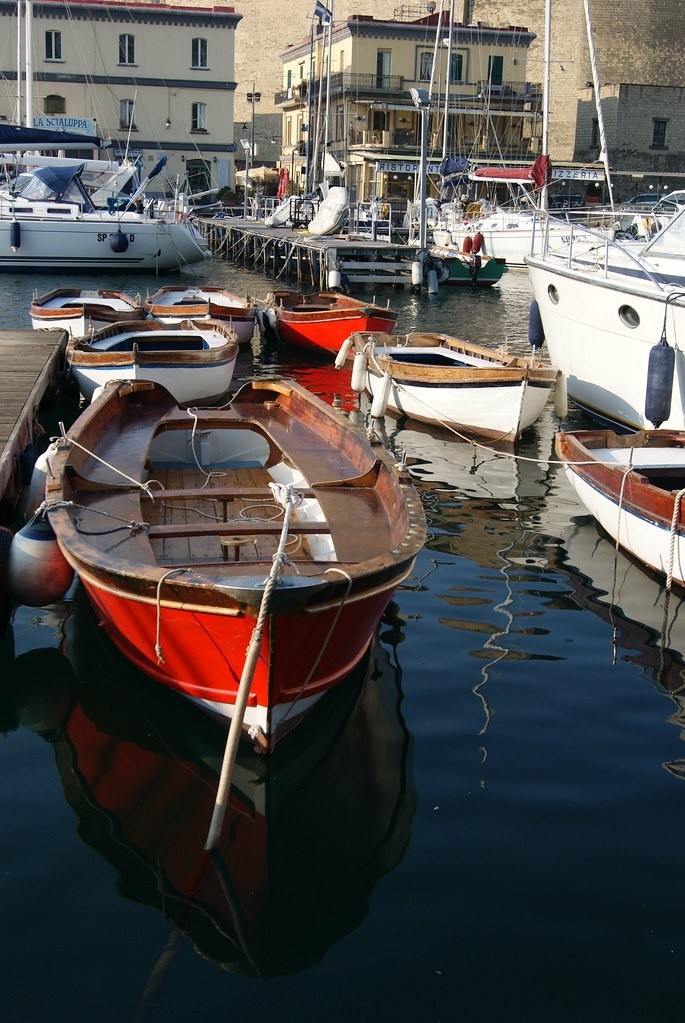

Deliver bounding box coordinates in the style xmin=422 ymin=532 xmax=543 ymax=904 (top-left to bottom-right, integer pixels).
xmin=350 ymin=329 xmax=562 ymax=445
xmin=27 ymin=286 xmax=143 ymax=340
xmin=431 ymin=210 xmax=616 ymax=266
xmin=64 ymin=316 xmax=240 ymax=407
xmin=265 ymin=288 xmax=399 ymax=362
xmin=144 ymin=285 xmax=258 ymax=345
xmin=520 ymin=203 xmax=684 ymax=436
xmin=0 ymin=89 xmax=216 ymax=274
xmin=552 ymin=425 xmax=685 ymax=589
xmin=42 ymin=370 xmax=432 ymax=756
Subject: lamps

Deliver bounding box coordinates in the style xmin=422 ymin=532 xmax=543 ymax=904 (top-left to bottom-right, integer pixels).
xmin=354 ymin=114 xmax=366 ymax=121
xmin=271 ymin=135 xmax=283 ymax=144
xmin=234 ymin=122 xmax=249 ymax=132
xmin=399 ymin=117 xmax=410 ymax=123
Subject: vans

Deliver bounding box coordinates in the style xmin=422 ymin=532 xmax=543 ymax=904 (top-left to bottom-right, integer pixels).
xmin=624 ymin=192 xmax=667 ymax=205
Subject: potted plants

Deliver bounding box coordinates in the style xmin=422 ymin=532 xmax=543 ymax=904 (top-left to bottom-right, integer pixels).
xmin=373 ymin=129 xmax=382 ymax=144
xmin=222 ymin=189 xmax=238 ymax=207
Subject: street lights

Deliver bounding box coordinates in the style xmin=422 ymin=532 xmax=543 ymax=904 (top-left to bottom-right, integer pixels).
xmin=408 ymin=86 xmax=433 ymax=249
xmin=239 ymin=139 xmax=252 ymax=219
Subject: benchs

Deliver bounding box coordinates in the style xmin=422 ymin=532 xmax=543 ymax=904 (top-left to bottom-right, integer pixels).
xmin=142 ymin=484 xmax=315 ymax=500
xmin=146 ymin=522 xmax=332 ymax=539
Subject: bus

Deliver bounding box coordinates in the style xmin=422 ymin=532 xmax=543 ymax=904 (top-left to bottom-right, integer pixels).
xmin=549 ymin=193 xmax=584 ymax=208
